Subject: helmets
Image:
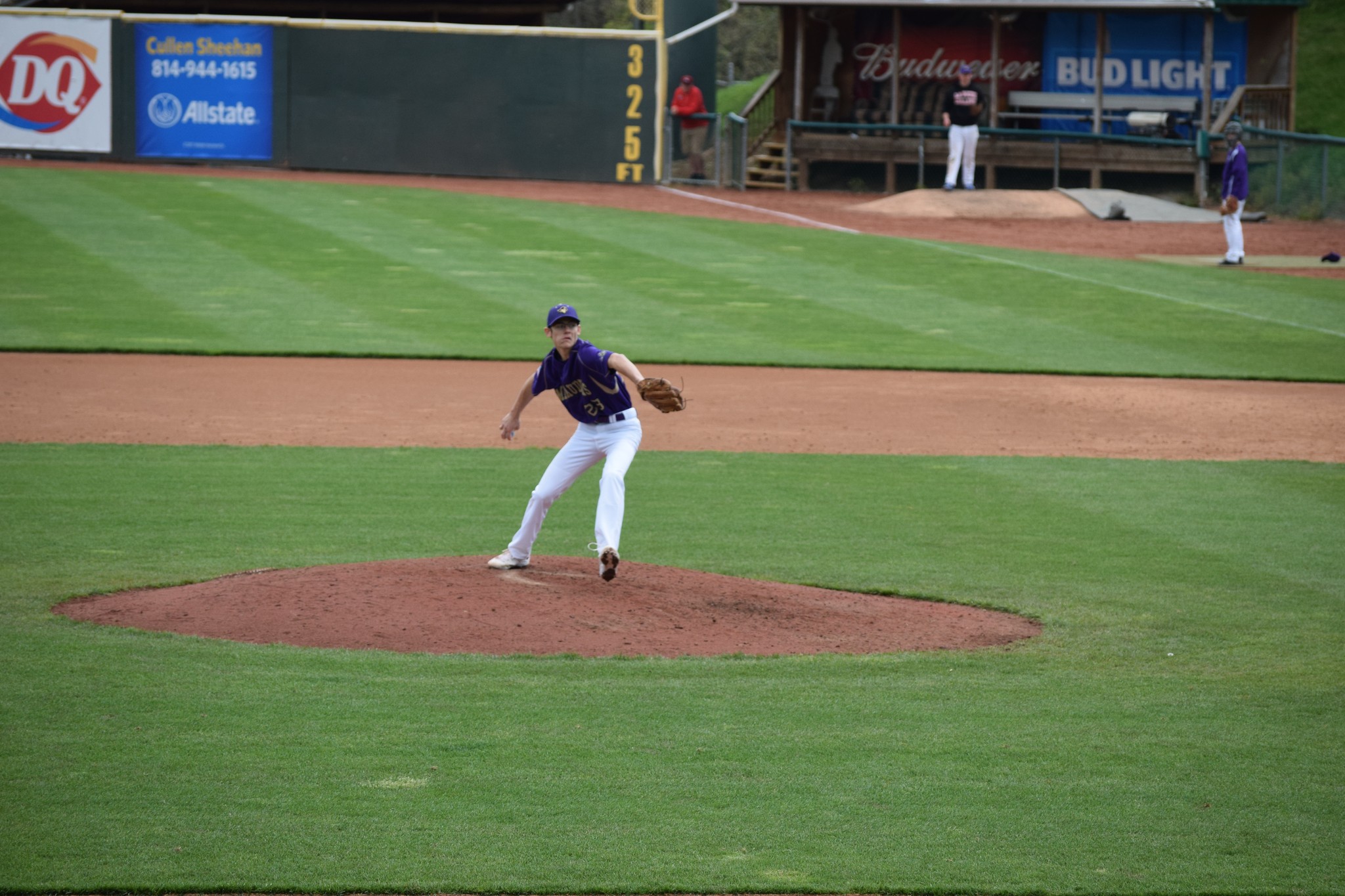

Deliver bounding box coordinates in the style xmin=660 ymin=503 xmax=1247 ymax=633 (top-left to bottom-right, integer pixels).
xmin=1224 ymin=121 xmax=1242 ymax=146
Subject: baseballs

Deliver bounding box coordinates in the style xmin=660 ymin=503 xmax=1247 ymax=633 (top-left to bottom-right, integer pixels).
xmin=503 ymin=427 xmax=515 ymax=439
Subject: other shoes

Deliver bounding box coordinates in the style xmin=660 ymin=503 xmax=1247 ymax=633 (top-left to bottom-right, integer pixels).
xmin=1221 ymin=258 xmax=1242 ymax=265
xmin=964 ymin=183 xmax=975 ymax=190
xmin=943 ymin=183 xmax=953 ymax=190
xmin=690 ymin=173 xmax=706 ymax=179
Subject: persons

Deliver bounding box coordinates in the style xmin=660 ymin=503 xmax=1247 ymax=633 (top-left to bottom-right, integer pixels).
xmin=1218 ymin=121 xmax=1248 ymax=265
xmin=669 ymin=74 xmax=708 ymax=181
xmin=942 ymin=66 xmax=986 ymax=190
xmin=486 ymin=303 xmax=685 ymax=582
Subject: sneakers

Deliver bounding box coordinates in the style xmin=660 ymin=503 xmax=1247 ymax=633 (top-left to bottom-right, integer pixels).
xmin=599 ymin=546 xmax=619 ymax=581
xmin=487 ymin=548 xmax=530 ymax=569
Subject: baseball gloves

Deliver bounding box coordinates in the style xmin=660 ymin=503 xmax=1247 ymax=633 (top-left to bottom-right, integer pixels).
xmin=636 ymin=378 xmax=686 ymax=414
xmin=1219 ymin=195 xmax=1238 ymax=215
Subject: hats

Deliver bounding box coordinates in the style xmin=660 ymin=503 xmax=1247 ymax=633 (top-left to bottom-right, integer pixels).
xmin=1322 ymin=252 xmax=1340 ymax=263
xmin=960 ymin=65 xmax=971 ymax=73
xmin=681 ymin=75 xmax=693 ymax=84
xmin=547 ymin=304 xmax=580 ymax=328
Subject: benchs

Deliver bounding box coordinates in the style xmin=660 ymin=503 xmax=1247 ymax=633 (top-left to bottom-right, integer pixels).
xmin=1009 ymin=91 xmax=1196 ymax=139
xmin=1193 ymin=98 xmax=1275 ymax=139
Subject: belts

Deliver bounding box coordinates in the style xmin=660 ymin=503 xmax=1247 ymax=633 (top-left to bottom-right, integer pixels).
xmin=594 ymin=414 xmax=624 ymax=423
